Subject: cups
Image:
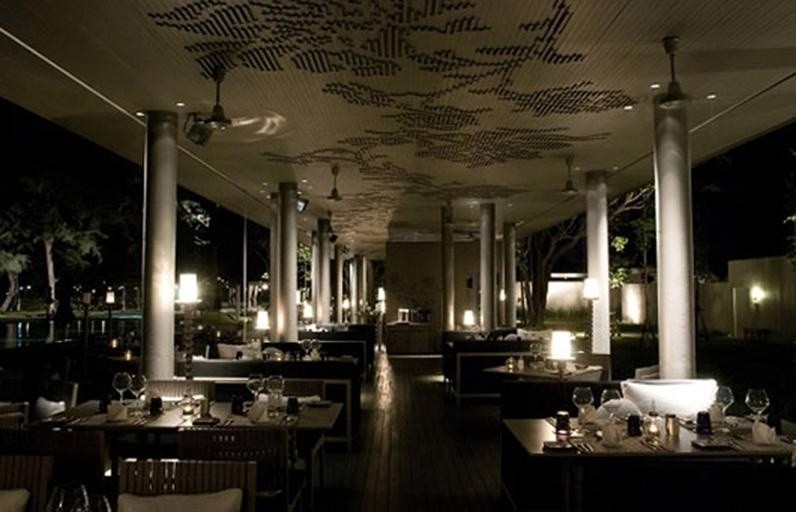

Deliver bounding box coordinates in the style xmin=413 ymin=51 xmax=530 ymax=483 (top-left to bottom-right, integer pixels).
xmin=695 ymin=410 xmax=713 ymax=434
xmin=231 ymin=394 xmax=245 ymax=414
xmin=628 ymin=414 xmax=642 ymax=436
xmin=555 ymin=410 xmax=571 ymax=435
xmin=286 ymin=397 xmax=301 ymax=415
xmin=150 ymin=396 xmax=164 ymax=416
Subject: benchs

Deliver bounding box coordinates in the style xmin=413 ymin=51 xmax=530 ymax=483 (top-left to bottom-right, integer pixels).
xmin=180 ymin=323 xmax=377 ymax=439
xmin=441 ymin=328 xmax=531 ymax=415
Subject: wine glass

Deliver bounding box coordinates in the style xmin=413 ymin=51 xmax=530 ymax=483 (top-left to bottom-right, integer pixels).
xmin=301 ymin=337 xmax=321 ymax=361
xmin=529 ymin=342 xmax=549 ymax=364
xmin=572 ymin=386 xmax=595 ymax=424
xmin=599 ymin=386 xmax=625 ymax=426
xmin=266 ymin=375 xmax=285 ymax=412
xmin=129 ymin=372 xmax=151 ymax=416
xmin=745 ymin=386 xmax=772 ymax=422
xmin=246 ymin=373 xmax=265 ymax=405
xmin=112 ymin=370 xmax=132 ymax=407
xmin=716 ymin=384 xmax=735 ymax=428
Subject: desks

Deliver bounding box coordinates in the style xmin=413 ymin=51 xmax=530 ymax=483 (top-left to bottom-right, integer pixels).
xmin=479 ymin=361 xmax=795 ymax=511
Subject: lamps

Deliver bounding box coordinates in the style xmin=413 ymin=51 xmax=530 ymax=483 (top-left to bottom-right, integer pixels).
xmin=561 ymin=155 xmax=581 ymax=195
xmin=326 ymin=166 xmax=343 ymax=203
xmin=206 ymin=66 xmax=232 ymax=132
xmin=657 ymin=36 xmax=691 ymax=110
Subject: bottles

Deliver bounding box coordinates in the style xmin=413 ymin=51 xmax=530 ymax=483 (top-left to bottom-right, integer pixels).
xmin=506 ymin=354 xmax=526 ymax=370
xmin=646 ymin=410 xmax=662 ymax=434
xmin=665 ymin=414 xmax=681 ymax=436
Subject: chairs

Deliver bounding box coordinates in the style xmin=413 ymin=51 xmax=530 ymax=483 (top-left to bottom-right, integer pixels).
xmin=0 ymin=427 xmax=294 ymax=510
